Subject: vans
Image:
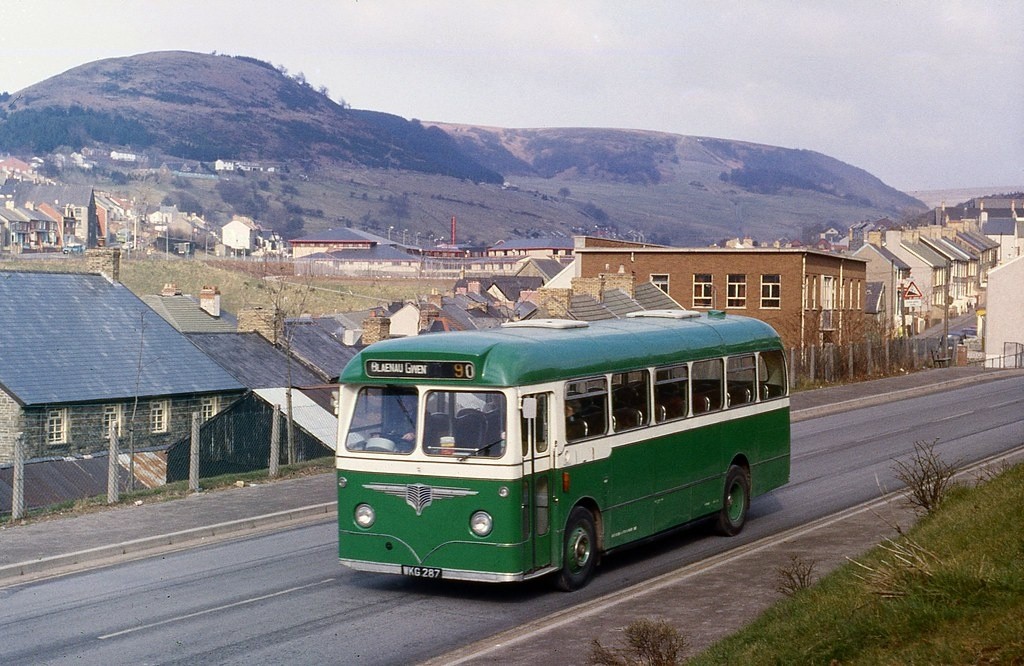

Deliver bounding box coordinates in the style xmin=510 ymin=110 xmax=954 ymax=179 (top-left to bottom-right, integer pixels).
xmin=936 ymin=331 xmax=966 ymax=353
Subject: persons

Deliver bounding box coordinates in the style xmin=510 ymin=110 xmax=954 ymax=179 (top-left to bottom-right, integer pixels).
xmin=566 ymin=400 xmax=585 ymax=440
xmin=372 ymin=394 xmax=418 ymax=442
xmin=482 ymin=393 xmax=505 ymax=413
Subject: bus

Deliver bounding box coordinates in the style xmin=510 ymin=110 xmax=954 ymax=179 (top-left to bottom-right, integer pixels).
xmin=336 ymin=310 xmax=791 ymax=594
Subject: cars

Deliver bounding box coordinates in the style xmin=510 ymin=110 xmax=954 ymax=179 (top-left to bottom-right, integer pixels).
xmin=961 ymin=328 xmax=977 ymax=339
xmin=63 ymin=244 xmax=82 ymax=254
xmin=967 ymin=324 xmax=977 ymax=328
